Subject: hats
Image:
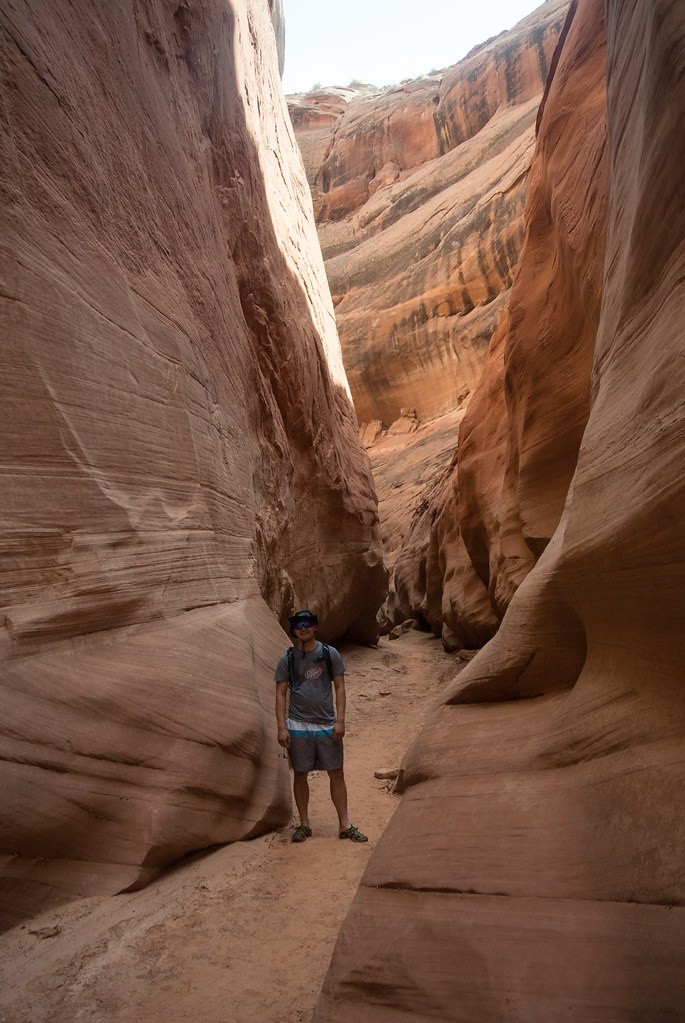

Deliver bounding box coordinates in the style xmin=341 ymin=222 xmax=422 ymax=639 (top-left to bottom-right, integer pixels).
xmin=288 ymin=610 xmax=318 ymax=622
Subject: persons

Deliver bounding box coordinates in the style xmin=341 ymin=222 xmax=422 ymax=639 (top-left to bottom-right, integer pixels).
xmin=274 ymin=610 xmax=368 ymax=842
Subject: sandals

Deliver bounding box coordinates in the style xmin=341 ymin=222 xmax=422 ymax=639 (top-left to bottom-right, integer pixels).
xmin=291 ymin=826 xmax=312 ymax=842
xmin=338 ymin=824 xmax=368 ymax=842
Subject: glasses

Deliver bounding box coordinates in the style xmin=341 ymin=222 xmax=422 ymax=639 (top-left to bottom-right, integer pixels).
xmin=294 ymin=622 xmax=314 ymax=630
